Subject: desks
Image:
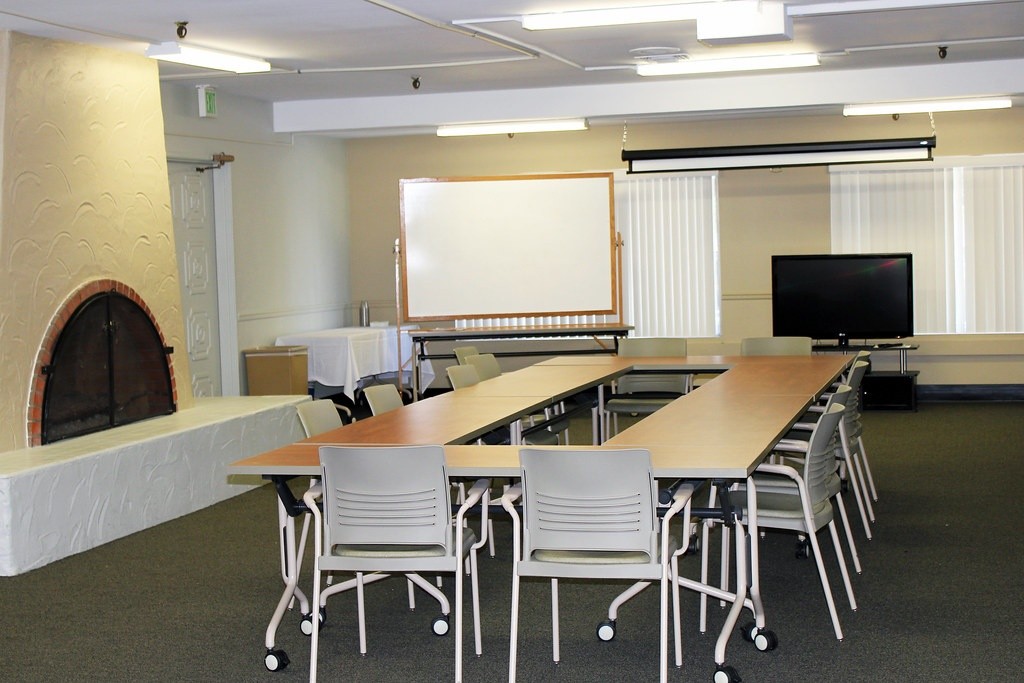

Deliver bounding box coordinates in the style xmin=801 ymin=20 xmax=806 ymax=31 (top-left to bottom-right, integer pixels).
xmin=407 ymin=322 xmax=634 ymax=402
xmin=232 ymin=354 xmax=856 ymax=683
xmin=274 ymin=325 xmax=436 ymax=422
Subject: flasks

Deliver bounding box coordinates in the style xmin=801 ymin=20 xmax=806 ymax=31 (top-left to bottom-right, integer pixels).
xmin=359 ymin=300 xmax=370 ymax=327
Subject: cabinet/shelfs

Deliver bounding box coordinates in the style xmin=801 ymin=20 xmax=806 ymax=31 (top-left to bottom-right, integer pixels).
xmin=812 ymin=343 xmax=921 ymax=412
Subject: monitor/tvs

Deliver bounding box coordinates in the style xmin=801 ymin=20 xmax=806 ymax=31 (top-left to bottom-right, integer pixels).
xmin=772 ymin=252 xmax=913 ymax=349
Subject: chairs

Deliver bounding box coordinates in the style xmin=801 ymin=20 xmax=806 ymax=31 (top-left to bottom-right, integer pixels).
xmin=303 ymin=442 xmax=489 ymax=683
xmin=500 ymin=445 xmax=696 ymax=683
xmin=698 ymin=349 xmax=882 ymax=643
xmin=293 ymin=345 xmax=569 ymax=614
